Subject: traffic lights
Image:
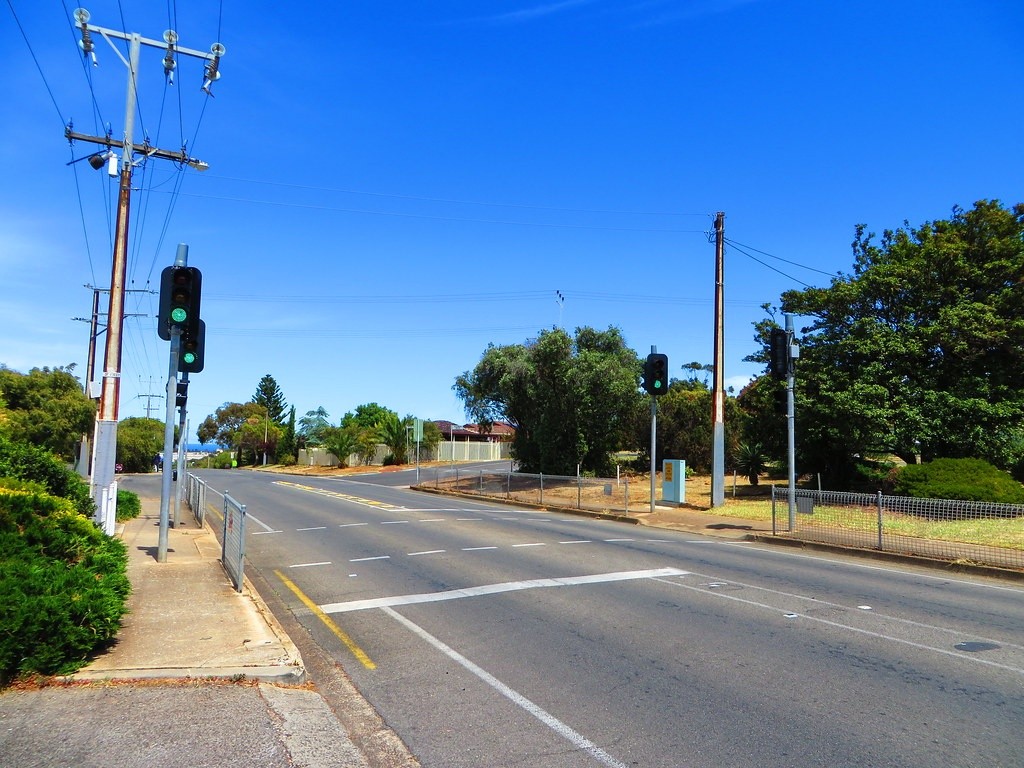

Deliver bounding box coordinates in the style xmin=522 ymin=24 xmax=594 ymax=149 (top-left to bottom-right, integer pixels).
xmin=646 ymin=353 xmax=670 ymax=396
xmin=765 ymin=328 xmax=788 ymax=381
xmin=158 ymin=265 xmax=202 ymax=343
xmin=175 ymin=319 xmax=206 ymax=373
xmin=641 ymin=362 xmax=648 ymax=392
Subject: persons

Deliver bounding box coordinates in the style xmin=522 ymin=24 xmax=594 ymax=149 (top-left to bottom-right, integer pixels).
xmin=152 ymin=453 xmax=161 ymax=472
xmin=161 ymin=454 xmax=164 ymax=472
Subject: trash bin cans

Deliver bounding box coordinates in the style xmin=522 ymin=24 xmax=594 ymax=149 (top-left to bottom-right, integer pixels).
xmin=173 ymin=469 xmax=177 ymax=481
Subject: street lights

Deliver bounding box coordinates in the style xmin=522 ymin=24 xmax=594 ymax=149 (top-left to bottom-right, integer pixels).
xmin=86 ymin=147 xmax=144 ymax=526
